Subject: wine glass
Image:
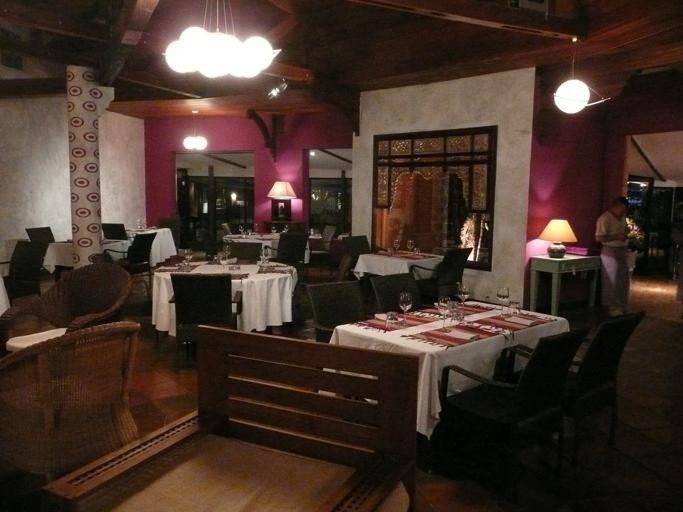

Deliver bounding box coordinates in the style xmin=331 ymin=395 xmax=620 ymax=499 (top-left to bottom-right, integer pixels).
xmin=387 ymin=238 xmax=420 ymax=257
xmin=384 ymin=279 xmax=521 ymax=333
xmin=238 ymin=221 xmax=315 ymax=239
xmin=174 ymin=244 xmax=269 ymax=272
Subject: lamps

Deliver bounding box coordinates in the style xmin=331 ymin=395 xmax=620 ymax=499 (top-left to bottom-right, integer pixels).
xmin=180 ymin=109 xmax=212 ymax=153
xmin=266 ymin=179 xmax=298 ymax=220
xmin=538 ymin=219 xmax=578 ymax=258
xmin=159 ymin=0 xmax=282 ymax=85
xmin=552 ymin=32 xmax=592 ymax=118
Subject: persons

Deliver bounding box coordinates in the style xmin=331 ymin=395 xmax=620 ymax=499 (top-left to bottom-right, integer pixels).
xmin=595 ymin=195 xmax=630 ymax=316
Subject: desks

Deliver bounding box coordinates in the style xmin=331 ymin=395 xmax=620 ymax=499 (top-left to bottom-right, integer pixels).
xmin=335 ymin=301 xmax=568 ymax=474
xmin=529 ymin=255 xmax=601 ymax=316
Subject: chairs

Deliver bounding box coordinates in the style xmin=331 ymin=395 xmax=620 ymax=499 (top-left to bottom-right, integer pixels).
xmin=0 ymin=223 xmax=176 ymax=314
xmin=434 ymin=328 xmax=590 ymax=511
xmin=153 ymin=224 xmax=474 ymax=359
xmin=41 ymin=325 xmax=419 ymax=511
xmin=494 ymin=312 xmax=644 ymax=467
xmin=0 ymin=262 xmax=133 ymax=359
xmin=1 ymin=323 xmax=141 ymax=512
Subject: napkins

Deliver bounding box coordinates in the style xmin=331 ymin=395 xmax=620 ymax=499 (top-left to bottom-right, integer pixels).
xmin=444 ymin=329 xmax=480 ymax=341
xmin=505 ymin=316 xmax=536 ymax=327
